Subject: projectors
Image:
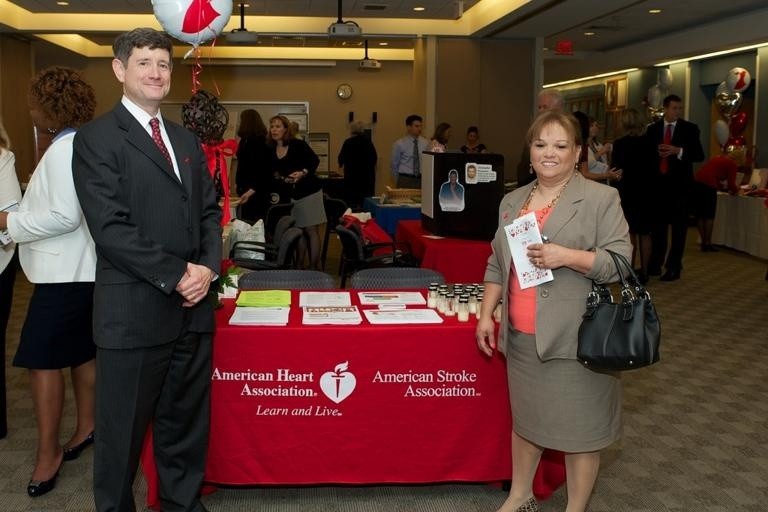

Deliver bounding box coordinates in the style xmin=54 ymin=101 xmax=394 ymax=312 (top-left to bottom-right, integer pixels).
xmin=360 ymin=59 xmax=382 ymax=69
xmin=226 ymin=31 xmax=257 ymax=43
xmin=329 ymin=23 xmax=361 ymax=44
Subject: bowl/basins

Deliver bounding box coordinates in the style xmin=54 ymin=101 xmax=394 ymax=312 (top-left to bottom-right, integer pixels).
xmin=221 ymin=197 xmax=241 ymax=208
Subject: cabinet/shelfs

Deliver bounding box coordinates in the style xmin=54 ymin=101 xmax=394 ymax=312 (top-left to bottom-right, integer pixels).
xmin=421 ymin=150 xmax=506 ymax=242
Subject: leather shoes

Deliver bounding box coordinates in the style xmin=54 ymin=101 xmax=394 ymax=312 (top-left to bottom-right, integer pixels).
xmin=659 ymin=268 xmax=680 ymax=281
xmin=619 ymin=262 xmax=662 ymax=286
xmin=27 ymin=447 xmax=66 ymax=498
xmin=701 ymin=242 xmax=721 ymax=252
xmin=512 ymin=494 xmax=539 ymax=512
xmin=62 ymin=430 xmax=94 ymax=461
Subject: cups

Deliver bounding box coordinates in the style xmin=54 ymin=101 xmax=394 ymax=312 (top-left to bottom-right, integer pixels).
xmin=657 ymin=144 xmax=669 ymax=157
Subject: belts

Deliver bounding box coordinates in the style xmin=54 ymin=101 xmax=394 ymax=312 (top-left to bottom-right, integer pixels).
xmin=399 ymin=172 xmax=422 ymax=179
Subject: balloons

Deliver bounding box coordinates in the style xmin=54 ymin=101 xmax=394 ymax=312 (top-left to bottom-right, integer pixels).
xmin=151 ymin=0 xmax=234 ymax=56
xmin=715 ymin=68 xmax=751 ymax=154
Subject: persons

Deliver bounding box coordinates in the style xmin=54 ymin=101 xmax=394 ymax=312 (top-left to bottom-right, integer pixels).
xmin=439 ymin=169 xmax=465 ymax=211
xmin=230 ymin=107 xmax=328 ymax=271
xmin=476 ymin=112 xmax=636 ymax=511
xmin=467 ymin=166 xmax=477 ymax=184
xmin=0 ymin=67 xmax=97 ymax=497
xmin=338 ymin=120 xmax=378 ymax=205
xmin=431 ymin=123 xmax=452 ymax=153
xmin=0 ymin=123 xmax=23 ymax=438
xmin=390 ymin=115 xmax=428 ymax=189
xmin=572 ymin=95 xmax=754 ymax=282
xmin=460 ymin=126 xmax=487 ymax=154
xmin=71 ymin=27 xmax=222 ymax=511
xmin=513 ymin=87 xmax=564 ymax=186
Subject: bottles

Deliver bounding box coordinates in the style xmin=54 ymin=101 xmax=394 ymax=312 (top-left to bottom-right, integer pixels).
xmin=427 ymin=282 xmax=503 ymax=325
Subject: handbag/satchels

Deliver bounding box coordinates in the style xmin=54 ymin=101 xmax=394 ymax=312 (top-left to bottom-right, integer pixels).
xmin=575 ymin=249 xmax=662 ymax=371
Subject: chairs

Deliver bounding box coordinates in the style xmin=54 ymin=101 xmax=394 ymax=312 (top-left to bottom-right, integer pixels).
xmin=231 ymin=190 xmax=442 ymax=292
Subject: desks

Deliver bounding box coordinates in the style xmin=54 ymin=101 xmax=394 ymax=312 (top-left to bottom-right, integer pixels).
xmin=685 ymin=191 xmax=767 ymax=261
xmin=140 ymin=288 xmax=569 ymax=511
xmin=219 ymin=192 xmax=241 ymax=264
xmin=398 ymin=216 xmax=494 ymax=283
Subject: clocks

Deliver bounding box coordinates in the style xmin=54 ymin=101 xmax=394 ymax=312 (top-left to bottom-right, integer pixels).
xmin=337 ymin=83 xmax=353 ymax=99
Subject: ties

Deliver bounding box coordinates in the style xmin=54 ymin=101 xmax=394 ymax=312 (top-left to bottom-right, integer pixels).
xmin=412 ymin=139 xmax=420 ymax=178
xmin=148 ymin=117 xmax=175 ymax=176
xmin=659 ymin=124 xmax=673 ymax=175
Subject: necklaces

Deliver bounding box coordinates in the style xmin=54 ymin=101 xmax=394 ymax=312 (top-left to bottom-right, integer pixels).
xmin=521 ymin=177 xmax=572 ymax=227
